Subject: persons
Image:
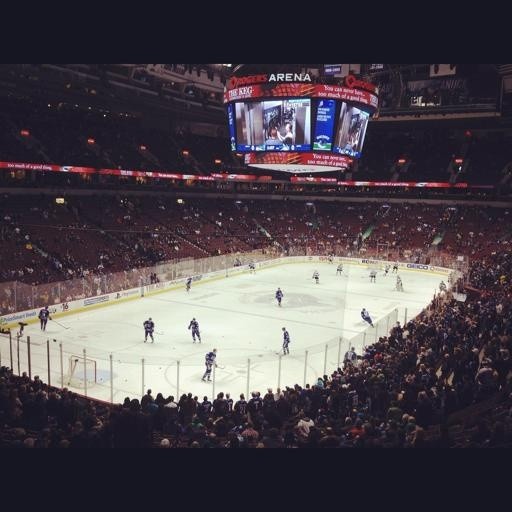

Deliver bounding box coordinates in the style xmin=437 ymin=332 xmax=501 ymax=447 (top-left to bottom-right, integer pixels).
xmin=2 ymin=108 xmax=512 ymax=451
